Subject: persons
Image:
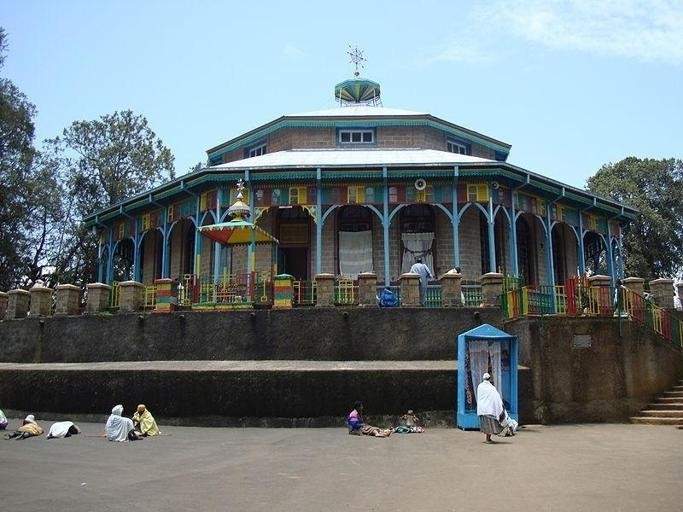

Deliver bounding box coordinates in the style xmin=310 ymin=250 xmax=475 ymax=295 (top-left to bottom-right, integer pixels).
xmin=0 ymin=410 xmax=8 ymax=430
xmin=410 ymin=256 xmax=433 ymax=307
xmin=132 ymin=403 xmax=160 ymax=437
xmin=447 ymin=266 xmax=465 ymax=306
xmin=46 ymin=425 xmax=78 ymax=439
xmin=614 ymin=278 xmax=626 ymax=314
xmin=5 ymin=414 xmax=36 ymax=440
xmin=348 ymin=401 xmax=392 ymax=437
xmin=105 ymin=404 xmax=143 ymax=443
xmin=476 ymin=371 xmax=505 ymax=444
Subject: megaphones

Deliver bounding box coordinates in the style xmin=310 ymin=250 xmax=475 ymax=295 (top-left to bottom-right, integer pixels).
xmin=490 ymin=181 xmax=499 ymax=189
xmin=414 ymin=178 xmax=426 ymax=191
